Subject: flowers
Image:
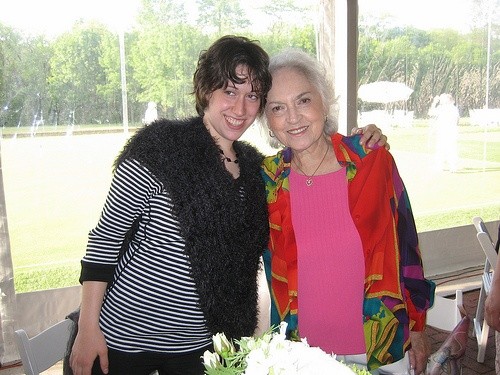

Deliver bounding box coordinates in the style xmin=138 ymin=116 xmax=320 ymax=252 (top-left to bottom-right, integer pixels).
xmin=200 ymin=320 xmax=372 ymax=375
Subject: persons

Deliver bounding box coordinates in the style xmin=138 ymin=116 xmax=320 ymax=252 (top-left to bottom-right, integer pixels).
xmin=261 ymin=49 xmax=431 ymax=375
xmin=64 ymin=36 xmax=390 ymax=375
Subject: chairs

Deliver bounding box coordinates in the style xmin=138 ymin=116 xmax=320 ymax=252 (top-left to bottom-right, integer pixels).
xmin=469 ymin=108 xmax=500 ymax=129
xmin=472 ymin=216 xmax=500 ymax=363
xmin=13 ymin=307 xmax=82 ymax=375
xmin=377 ymin=289 xmax=463 ymax=375
xmin=357 ymin=109 xmax=417 ymax=135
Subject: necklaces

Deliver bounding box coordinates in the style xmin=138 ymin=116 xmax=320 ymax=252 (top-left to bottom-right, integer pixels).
xmin=221 ymin=149 xmax=240 ymax=166
xmin=291 ymin=144 xmax=330 ymax=187
